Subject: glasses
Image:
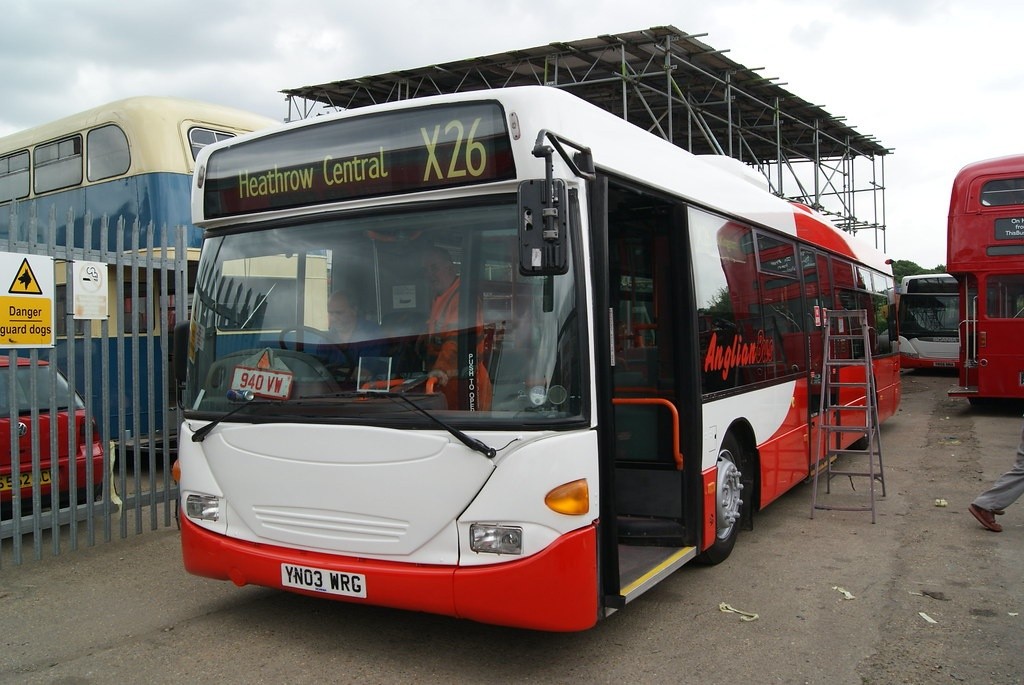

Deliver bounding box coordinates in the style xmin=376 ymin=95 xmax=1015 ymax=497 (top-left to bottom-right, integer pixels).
xmin=327 ymin=309 xmax=352 ymax=316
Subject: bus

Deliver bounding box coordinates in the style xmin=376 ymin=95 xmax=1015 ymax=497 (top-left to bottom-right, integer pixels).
xmin=169 ymin=83 xmax=902 ymax=632
xmin=0 ymin=92 xmax=330 ymax=452
xmin=895 ymin=273 xmax=961 ymax=371
xmin=944 ymin=151 xmax=1024 ymax=411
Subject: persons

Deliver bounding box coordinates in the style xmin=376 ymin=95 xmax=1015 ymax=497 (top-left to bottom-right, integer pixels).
xmin=419 ymin=247 xmax=495 ymax=411
xmin=968 ymin=433 xmax=1024 ymax=532
xmin=300 ymin=288 xmax=384 ymax=372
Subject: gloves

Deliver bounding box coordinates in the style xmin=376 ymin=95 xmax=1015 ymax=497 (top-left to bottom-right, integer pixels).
xmin=428 ymin=369 xmax=448 ymax=387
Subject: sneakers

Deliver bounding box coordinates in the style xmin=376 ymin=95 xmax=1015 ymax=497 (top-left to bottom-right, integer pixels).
xmin=969 ymin=503 xmax=1005 ymax=532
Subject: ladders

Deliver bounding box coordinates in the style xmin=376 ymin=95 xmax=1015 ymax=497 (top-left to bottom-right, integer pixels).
xmin=732 ymin=316 xmax=791 ymax=387
xmin=809 ymin=310 xmax=888 ymax=525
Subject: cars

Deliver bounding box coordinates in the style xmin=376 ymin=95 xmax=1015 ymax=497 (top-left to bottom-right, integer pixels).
xmin=0 ymin=352 xmax=104 ymax=513
